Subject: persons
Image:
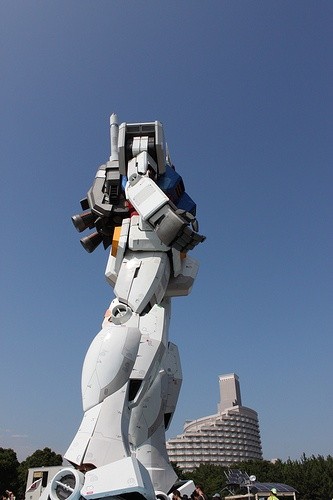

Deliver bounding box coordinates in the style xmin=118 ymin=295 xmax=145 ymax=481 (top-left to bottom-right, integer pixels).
xmin=172 ymin=483 xmax=212 ymax=500
xmin=266 ymin=488 xmax=279 ymax=500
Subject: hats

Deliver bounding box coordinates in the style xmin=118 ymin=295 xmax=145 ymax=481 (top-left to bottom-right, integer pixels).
xmin=268 ymin=488 xmax=277 ymax=495
